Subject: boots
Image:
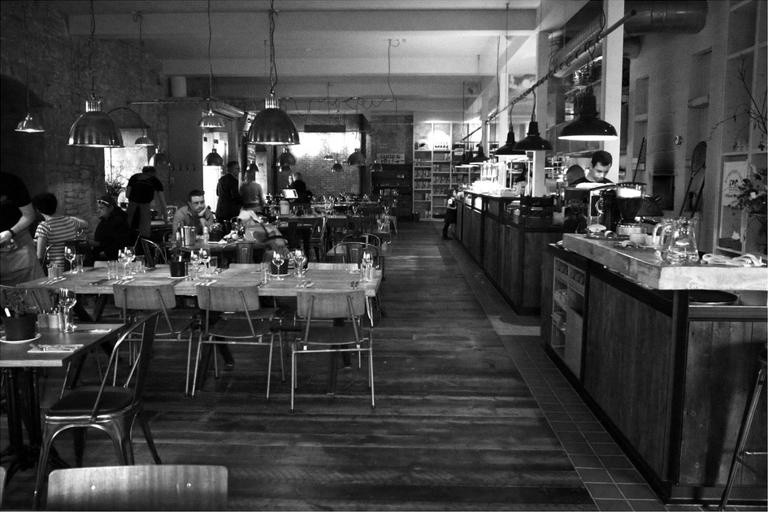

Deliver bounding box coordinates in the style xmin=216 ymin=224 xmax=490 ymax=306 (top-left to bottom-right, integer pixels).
xmin=443 ymin=229 xmax=452 ymax=240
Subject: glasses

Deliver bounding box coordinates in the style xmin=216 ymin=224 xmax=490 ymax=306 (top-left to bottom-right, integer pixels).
xmin=97 ymin=200 xmax=109 ymax=205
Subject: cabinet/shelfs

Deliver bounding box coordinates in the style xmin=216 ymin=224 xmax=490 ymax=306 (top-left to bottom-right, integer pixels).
xmin=549 ymin=257 xmax=586 ymax=383
xmin=412 ymin=121 xmax=474 ymax=221
xmin=713 ymin=0 xmax=767 ymax=258
xmin=371 ymin=164 xmax=411 ymax=198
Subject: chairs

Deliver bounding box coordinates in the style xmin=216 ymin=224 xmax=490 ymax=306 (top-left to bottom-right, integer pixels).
xmin=30 ymin=310 xmax=161 ymax=511
xmin=0 ymin=465 xmax=7 ymax=507
xmin=45 ymin=465 xmax=229 ymax=512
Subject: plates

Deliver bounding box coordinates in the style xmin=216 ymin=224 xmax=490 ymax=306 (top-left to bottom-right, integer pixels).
xmin=1 ymin=331 xmax=43 ymax=346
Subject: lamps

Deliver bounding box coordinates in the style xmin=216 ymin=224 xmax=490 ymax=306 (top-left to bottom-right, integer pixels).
xmin=242 ymin=0 xmax=301 ymax=147
xmin=66 ymin=0 xmax=124 ymax=149
xmin=13 ymin=0 xmax=47 ymax=132
xmin=324 ymin=96 xmax=385 ymax=174
xmin=203 ymin=132 xmax=227 ymax=165
xmin=453 ymin=130 xmax=488 ymax=170
xmin=273 ymin=146 xmax=297 ymax=173
xmin=559 ymin=51 xmax=618 ymax=141
xmin=147 ymin=140 xmax=172 ymax=176
xmin=491 ymin=105 xmax=523 ymax=153
xmin=199 ymin=0 xmax=229 ymax=130
xmin=134 ymin=11 xmax=153 ymax=147
xmin=512 ymin=86 xmax=555 ymax=152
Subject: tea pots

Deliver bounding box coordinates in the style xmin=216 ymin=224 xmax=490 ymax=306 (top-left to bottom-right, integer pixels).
xmin=651 ymin=219 xmax=699 ymax=265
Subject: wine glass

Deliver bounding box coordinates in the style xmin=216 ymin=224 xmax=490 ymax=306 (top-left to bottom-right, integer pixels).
xmin=65 ymin=246 xmax=77 ymax=274
xmin=124 ymin=247 xmax=136 ymax=278
xmin=191 ymin=250 xmax=203 ymax=281
xmin=118 ymin=249 xmax=130 ymax=282
xmin=59 ymin=288 xmax=77 ymax=334
xmin=200 ymin=248 xmax=211 ymax=276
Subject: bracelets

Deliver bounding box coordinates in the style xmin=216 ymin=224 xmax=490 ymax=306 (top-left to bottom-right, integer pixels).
xmin=7 ymin=229 xmax=16 ymax=239
xmin=198 ymin=215 xmax=204 ymax=219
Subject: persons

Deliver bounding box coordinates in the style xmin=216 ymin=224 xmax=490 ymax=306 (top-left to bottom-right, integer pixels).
xmin=214 ymin=159 xmax=307 ymax=256
xmin=566 ymin=165 xmax=585 ymax=186
xmin=172 ymin=189 xmax=224 ymax=269
xmin=0 ymin=175 xmax=45 ymax=287
xmin=442 ymin=189 xmax=457 ymax=240
xmin=32 ymin=192 xmax=89 ymax=278
xmin=564 ymin=150 xmax=614 ymax=219
xmin=125 ymin=165 xmax=168 ymax=254
xmin=83 ymin=195 xmax=129 ymax=266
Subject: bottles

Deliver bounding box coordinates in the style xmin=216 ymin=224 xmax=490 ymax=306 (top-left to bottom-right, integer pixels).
xmin=272 ymin=248 xmax=307 ymax=281
